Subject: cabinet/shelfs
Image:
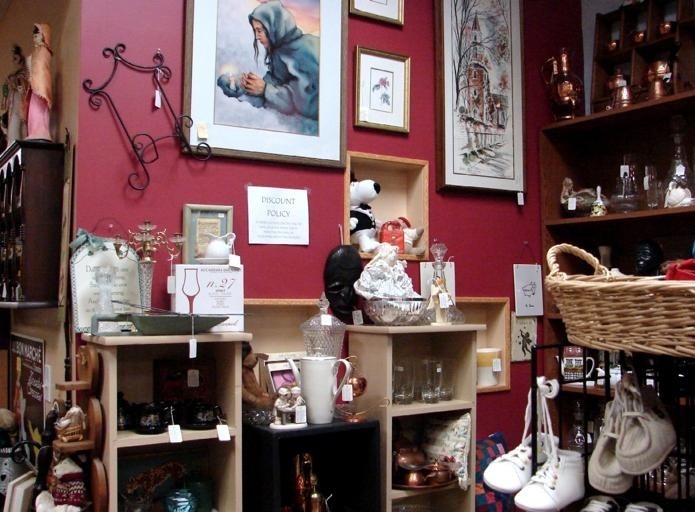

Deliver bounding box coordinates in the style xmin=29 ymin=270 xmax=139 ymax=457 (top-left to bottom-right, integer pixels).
xmin=87 ymin=334 xmax=253 ymax=512
xmin=0 ymin=139 xmax=67 ymax=308
xmin=538 ymin=1 xmax=695 ymax=512
xmin=344 ymin=324 xmax=487 ymax=512
xmin=49 ymin=381 xmax=95 ymax=457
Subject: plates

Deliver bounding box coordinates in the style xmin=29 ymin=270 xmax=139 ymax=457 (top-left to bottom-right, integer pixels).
xmin=195 ymin=257 xmax=228 ymax=265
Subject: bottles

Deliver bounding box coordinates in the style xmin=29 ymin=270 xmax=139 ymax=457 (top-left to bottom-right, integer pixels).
xmin=566 ymin=408 xmax=594 ymax=453
xmin=298 ymin=453 xmax=321 ymax=512
xmin=299 ymin=292 xmax=345 ymax=375
xmin=589 ymin=127 xmax=692 ymax=215
xmin=418 ymin=242 xmax=466 ymax=324
xmin=392 ymin=351 xmax=458 ymax=406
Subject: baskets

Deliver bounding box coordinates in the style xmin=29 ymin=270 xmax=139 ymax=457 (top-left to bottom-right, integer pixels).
xmin=543 ymin=243 xmax=694 ymax=358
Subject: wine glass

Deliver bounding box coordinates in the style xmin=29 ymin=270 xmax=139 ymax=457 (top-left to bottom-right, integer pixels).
xmin=182 ymin=268 xmax=200 ymax=314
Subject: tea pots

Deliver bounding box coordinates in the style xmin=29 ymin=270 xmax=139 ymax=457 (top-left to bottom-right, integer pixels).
xmin=605 ymin=59 xmax=671 ymax=111
xmin=203 ymin=231 xmax=235 ymax=258
xmin=540 ymin=48 xmax=584 ymax=120
xmin=392 ymin=424 xmax=453 ymax=486
xmin=555 ymin=355 xmax=595 ymax=380
xmin=282 ymin=349 xmax=350 ymax=422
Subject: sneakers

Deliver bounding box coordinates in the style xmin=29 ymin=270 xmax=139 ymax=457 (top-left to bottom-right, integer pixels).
xmin=483 ymin=374 xmax=676 ymax=511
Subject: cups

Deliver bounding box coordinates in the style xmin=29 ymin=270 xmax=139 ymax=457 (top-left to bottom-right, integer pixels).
xmin=477 ymin=347 xmax=502 ymax=388
xmin=117 ymin=392 xmax=224 ymax=435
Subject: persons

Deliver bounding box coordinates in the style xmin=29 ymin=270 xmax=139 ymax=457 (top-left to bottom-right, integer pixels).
xmin=2 ymin=43 xmax=31 ymax=148
xmin=26 ymin=21 xmax=56 ymax=143
xmin=241 ymin=0 xmax=318 ymax=121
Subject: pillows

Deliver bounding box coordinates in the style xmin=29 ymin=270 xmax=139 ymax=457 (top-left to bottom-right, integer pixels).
xmin=476 ymin=431 xmax=512 ymax=511
xmin=419 ymin=414 xmax=473 ymax=490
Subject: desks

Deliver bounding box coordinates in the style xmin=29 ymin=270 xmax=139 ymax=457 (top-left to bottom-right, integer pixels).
xmin=242 ymin=417 xmax=380 ymax=512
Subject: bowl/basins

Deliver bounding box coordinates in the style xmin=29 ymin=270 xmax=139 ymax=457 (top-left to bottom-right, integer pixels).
xmin=128 ymin=314 xmax=230 ymax=336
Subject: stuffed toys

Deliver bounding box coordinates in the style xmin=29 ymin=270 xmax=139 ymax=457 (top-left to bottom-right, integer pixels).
xmin=397 ymin=216 xmax=426 ymax=256
xmin=243 ymin=342 xmax=279 ymax=410
xmin=350 ymin=169 xmax=381 ymax=254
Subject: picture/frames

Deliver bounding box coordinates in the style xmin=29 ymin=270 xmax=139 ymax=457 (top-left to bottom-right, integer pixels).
xmin=353 ymin=45 xmax=412 ymax=135
xmin=179 ymin=0 xmax=349 ymax=173
xmin=259 ymin=351 xmax=306 ymax=393
xmin=181 ymin=204 xmax=234 ymax=264
xmin=434 ymin=0 xmax=528 ymax=197
xmin=263 ymin=362 xmax=301 ymax=403
xmin=349 ymin=0 xmax=405 ymax=26
